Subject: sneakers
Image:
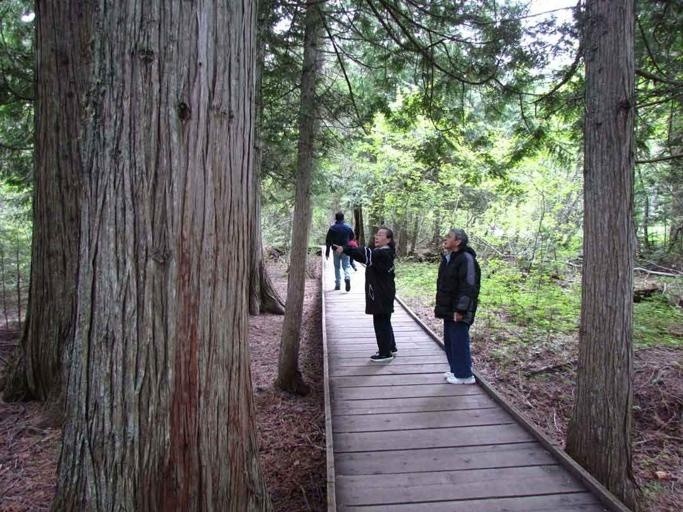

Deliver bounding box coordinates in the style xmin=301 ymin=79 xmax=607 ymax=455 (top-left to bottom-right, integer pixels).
xmin=370 ymin=347 xmax=397 ymax=362
xmin=444 ymin=372 xmax=476 ymax=385
xmin=335 ymin=279 xmax=350 ymax=291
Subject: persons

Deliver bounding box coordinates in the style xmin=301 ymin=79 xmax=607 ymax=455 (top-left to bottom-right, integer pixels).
xmin=326 ymin=211 xmax=363 ymax=292
xmin=433 ymin=228 xmax=481 ymax=384
xmin=333 ymin=227 xmax=400 ymax=363
